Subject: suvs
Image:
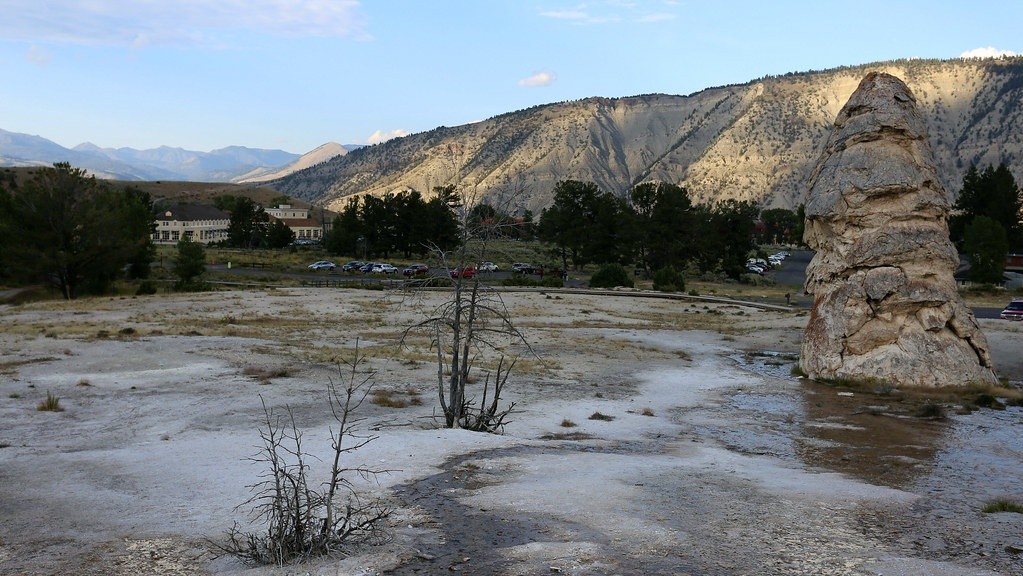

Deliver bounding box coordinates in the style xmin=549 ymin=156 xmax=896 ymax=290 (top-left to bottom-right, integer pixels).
xmin=342 ymin=260 xmax=399 ymax=274
xmin=402 ymin=264 xmax=429 ymax=276
xmin=474 ymin=262 xmax=500 ymax=272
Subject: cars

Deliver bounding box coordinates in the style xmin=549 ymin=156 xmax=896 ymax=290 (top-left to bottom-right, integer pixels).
xmin=293 ymin=239 xmax=316 ymax=245
xmin=1005 ymin=254 xmax=1023 ymax=267
xmin=307 ymin=260 xmax=336 ymax=271
xmin=511 ymin=263 xmax=539 ymax=275
xmin=1000 ymin=300 xmax=1023 ymax=321
xmin=745 ymin=250 xmax=789 ymax=274
xmin=450 ymin=267 xmax=476 ymax=279
